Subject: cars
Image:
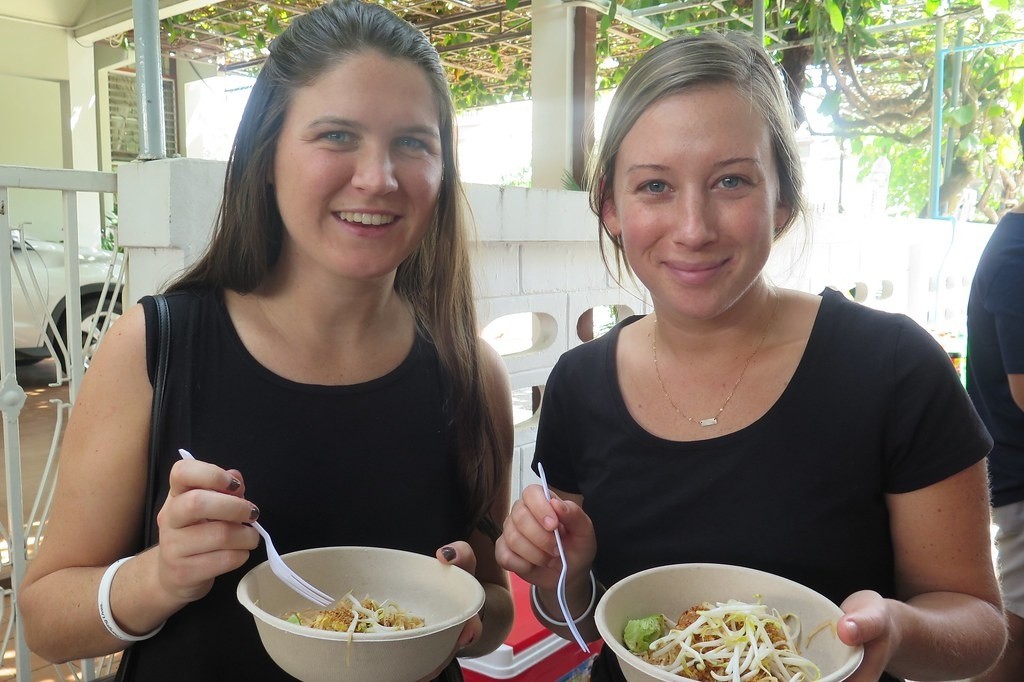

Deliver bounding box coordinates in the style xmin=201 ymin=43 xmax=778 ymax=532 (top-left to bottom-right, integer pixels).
xmin=2 ymin=225 xmax=127 ymax=380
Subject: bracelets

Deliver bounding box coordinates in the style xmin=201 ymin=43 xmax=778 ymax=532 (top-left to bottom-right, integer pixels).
xmin=98 ymin=555 xmax=166 ymax=641
xmin=532 ymin=570 xmax=596 ymax=626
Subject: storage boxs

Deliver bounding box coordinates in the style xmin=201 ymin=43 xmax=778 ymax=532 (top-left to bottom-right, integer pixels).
xmin=454 ymin=570 xmax=604 ymax=682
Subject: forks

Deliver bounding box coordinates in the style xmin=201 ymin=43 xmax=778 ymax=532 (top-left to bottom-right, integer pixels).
xmin=179 ymin=449 xmax=335 ymax=609
xmin=538 ymin=462 xmax=591 ymax=654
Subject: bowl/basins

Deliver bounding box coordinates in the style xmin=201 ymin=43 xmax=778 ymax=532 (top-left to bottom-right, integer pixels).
xmin=594 ymin=563 xmax=865 ymax=682
xmin=236 ymin=546 xmax=486 ymax=682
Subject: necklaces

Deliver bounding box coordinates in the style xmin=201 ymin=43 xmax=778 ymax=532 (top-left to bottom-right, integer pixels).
xmin=653 ymin=287 xmax=779 ymax=428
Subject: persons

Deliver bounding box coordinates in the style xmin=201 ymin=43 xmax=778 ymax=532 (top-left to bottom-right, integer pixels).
xmin=17 ymin=0 xmax=516 ymax=682
xmin=965 ymin=119 xmax=1024 ymax=682
xmin=495 ymin=33 xmax=1007 ymax=682
